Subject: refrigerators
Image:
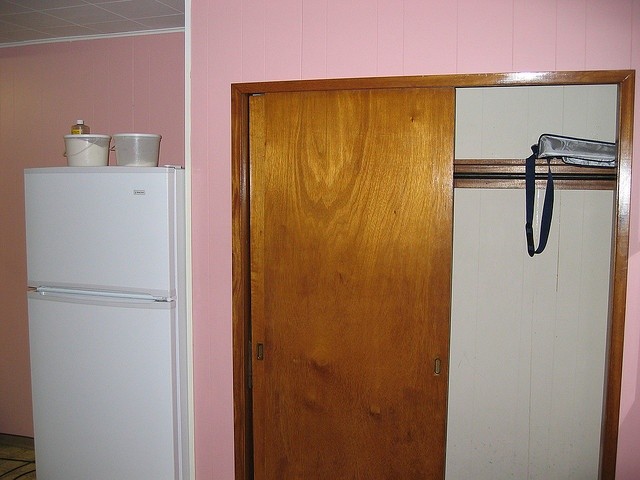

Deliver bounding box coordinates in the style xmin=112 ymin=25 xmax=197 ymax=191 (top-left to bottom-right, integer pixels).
xmin=24 ymin=162 xmax=185 ymax=476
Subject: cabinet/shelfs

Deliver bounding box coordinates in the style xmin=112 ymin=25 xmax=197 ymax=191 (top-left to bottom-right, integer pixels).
xmin=226 ymin=71 xmax=631 ymax=474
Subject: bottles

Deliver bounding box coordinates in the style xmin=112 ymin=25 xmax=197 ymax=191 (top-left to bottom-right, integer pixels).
xmin=71 ymin=120 xmax=90 ymax=134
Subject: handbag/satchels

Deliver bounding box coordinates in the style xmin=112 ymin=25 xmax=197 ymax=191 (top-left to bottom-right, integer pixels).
xmin=520 ymin=132 xmax=624 ymax=261
